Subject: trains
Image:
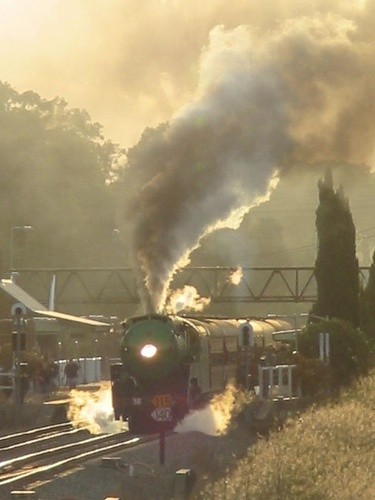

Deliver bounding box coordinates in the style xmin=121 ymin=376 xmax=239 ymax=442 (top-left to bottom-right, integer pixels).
xmin=110 ymin=314 xmax=299 ymax=434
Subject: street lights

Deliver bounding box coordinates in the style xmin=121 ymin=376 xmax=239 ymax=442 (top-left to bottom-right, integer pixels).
xmin=11 ymin=225 xmax=34 ymax=278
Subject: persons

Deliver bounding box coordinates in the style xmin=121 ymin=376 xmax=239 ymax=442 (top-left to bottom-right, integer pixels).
xmin=63 ymin=357 xmax=82 ymax=390
